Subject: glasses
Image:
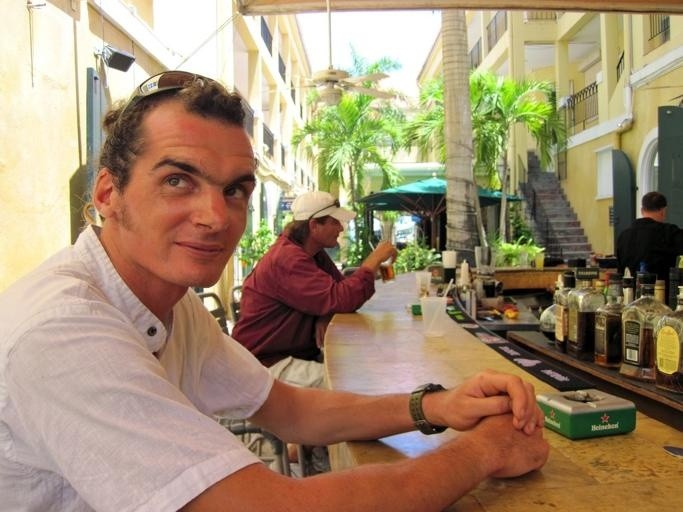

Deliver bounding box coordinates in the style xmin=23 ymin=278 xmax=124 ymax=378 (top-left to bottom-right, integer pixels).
xmin=305 ymin=198 xmax=340 ymax=224
xmin=116 ymin=70 xmax=230 ymax=129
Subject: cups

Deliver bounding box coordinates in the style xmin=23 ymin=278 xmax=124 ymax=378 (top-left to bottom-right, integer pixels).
xmin=417 ymin=272 xmax=430 ymax=295
xmin=482 ymin=296 xmax=504 ymax=308
xmin=380 ymin=258 xmax=397 ymax=284
xmin=475 ymin=246 xmax=495 ymax=273
xmin=443 ymin=267 xmax=457 ymax=284
xmin=420 ymin=297 xmax=447 ymax=337
xmin=534 ymin=253 xmax=544 ymax=268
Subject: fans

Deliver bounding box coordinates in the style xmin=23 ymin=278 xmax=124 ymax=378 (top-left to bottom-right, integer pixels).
xmin=268 ymin=0 xmax=397 ymax=106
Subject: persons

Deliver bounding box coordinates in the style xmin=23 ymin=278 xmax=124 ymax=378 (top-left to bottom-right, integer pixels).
xmin=614 ymin=190 xmax=683 ymax=305
xmin=230 ymin=190 xmax=399 ymax=463
xmin=1 ymin=70 xmax=552 ymax=512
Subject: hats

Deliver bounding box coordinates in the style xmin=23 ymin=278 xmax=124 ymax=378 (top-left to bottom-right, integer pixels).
xmin=290 ymin=190 xmax=357 ymax=222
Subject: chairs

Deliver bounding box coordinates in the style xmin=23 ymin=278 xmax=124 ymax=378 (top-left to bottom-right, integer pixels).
xmin=342 ymin=267 xmax=359 ymax=276
xmin=196 ymin=286 xmax=307 ymax=479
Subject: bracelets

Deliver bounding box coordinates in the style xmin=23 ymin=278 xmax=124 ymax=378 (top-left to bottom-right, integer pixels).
xmin=411 ymin=381 xmax=448 ymax=433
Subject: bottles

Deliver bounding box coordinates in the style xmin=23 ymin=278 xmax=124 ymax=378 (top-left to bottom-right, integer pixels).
xmin=540 ymin=254 xmax=683 ymax=394
xmin=461 ymin=285 xmax=478 ymax=320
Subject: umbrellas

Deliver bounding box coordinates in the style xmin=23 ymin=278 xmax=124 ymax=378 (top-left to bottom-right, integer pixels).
xmin=361 ymin=171 xmax=526 ymax=250
